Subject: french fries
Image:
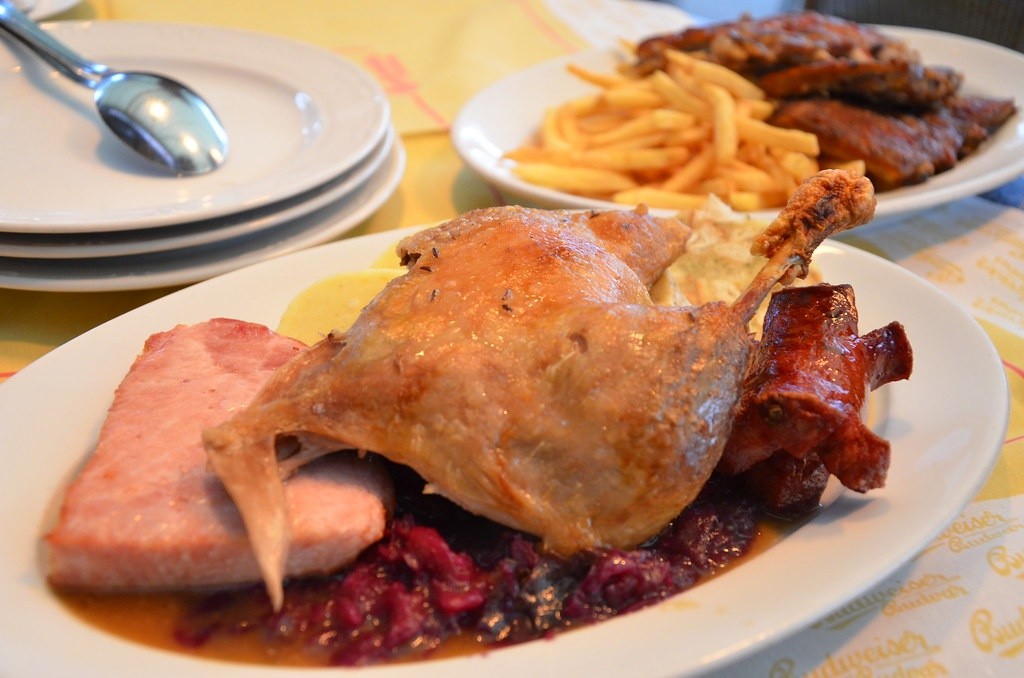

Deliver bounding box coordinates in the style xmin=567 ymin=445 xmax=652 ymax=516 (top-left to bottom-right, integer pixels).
xmin=499 ymin=36 xmax=873 ymax=211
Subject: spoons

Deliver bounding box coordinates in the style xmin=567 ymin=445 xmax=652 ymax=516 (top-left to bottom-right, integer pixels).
xmin=1 ymin=0 xmax=229 ymax=177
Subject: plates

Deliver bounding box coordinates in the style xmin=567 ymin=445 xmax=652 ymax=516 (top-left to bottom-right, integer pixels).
xmin=12 ymin=0 xmax=79 ymax=20
xmin=447 ymin=25 xmax=1024 ymax=232
xmin=0 ymin=20 xmax=404 ymax=287
xmin=2 ymin=208 xmax=1010 ymax=676
